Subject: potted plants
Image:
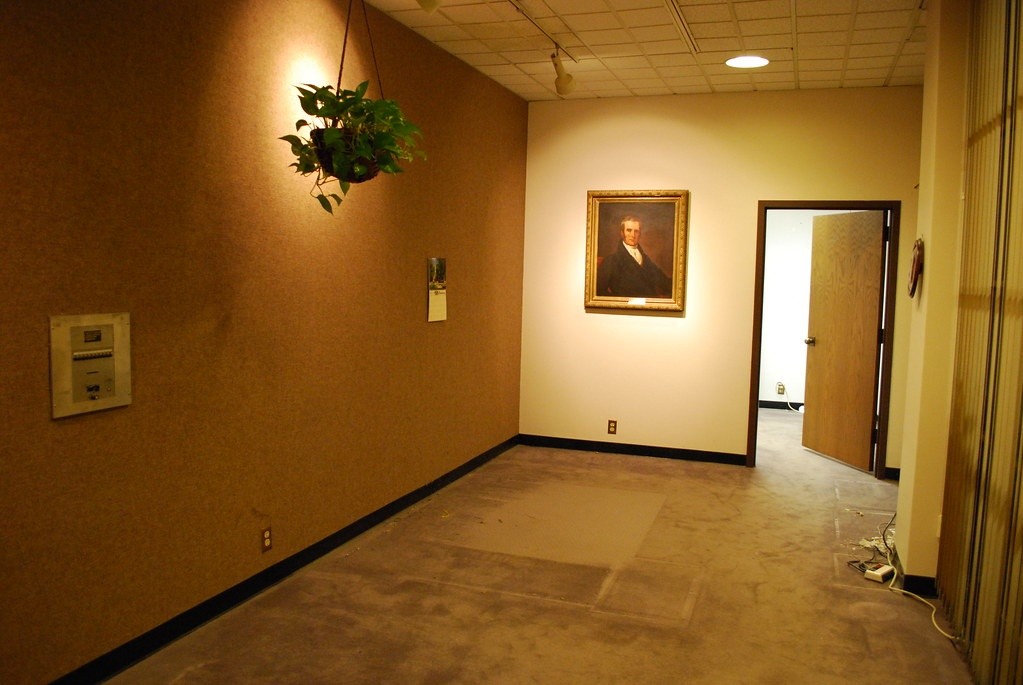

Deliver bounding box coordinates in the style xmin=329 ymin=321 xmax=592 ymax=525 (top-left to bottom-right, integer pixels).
xmin=277 ymin=83 xmax=429 ymax=217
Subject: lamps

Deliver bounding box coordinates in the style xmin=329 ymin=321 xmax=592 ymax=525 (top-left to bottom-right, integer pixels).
xmin=415 ymin=0 xmax=443 ymax=16
xmin=550 ymin=45 xmax=576 ymax=95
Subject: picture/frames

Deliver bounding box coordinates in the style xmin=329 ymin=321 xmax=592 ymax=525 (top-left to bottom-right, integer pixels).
xmin=584 ymin=189 xmax=690 ymax=313
